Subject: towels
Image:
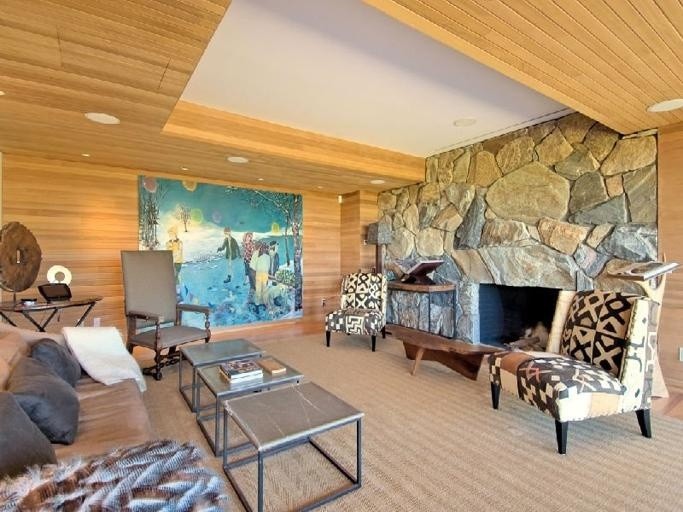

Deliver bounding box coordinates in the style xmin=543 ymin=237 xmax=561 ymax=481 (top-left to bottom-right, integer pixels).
xmin=60 ymin=326 xmax=147 ymax=392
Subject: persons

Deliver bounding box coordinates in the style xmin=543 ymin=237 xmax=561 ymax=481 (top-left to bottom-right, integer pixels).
xmin=216 ymin=227 xmax=279 ymax=314
xmin=160 ymin=230 xmax=196 ymax=306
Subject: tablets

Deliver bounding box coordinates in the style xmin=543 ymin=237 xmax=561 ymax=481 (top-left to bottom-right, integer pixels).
xmin=38 ymin=283 xmax=71 ymax=301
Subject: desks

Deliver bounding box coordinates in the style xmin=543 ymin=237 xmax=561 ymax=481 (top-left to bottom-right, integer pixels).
xmin=388 ymin=280 xmax=456 ymax=339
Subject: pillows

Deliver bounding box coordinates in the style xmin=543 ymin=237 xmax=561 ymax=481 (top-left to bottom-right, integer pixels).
xmin=6 ymin=356 xmax=79 ymax=445
xmin=0 ymin=389 xmax=59 ymax=481
xmin=30 ymin=338 xmax=82 ymax=388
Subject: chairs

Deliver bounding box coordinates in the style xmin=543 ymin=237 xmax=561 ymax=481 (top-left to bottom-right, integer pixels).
xmin=324 ymin=272 xmax=387 ymax=351
xmin=486 ymin=290 xmax=655 ymax=454
xmin=121 ymin=249 xmax=211 ymax=380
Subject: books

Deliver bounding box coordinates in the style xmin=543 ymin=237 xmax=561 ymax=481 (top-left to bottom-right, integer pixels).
xmin=218 ymin=358 xmax=285 ymax=381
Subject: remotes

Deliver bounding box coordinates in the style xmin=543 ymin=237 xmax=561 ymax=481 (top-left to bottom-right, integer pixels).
xmin=22 ymin=298 xmax=37 ymax=301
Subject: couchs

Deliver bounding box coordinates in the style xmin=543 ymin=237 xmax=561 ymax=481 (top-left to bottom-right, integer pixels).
xmin=0 ymin=324 xmax=221 ymax=512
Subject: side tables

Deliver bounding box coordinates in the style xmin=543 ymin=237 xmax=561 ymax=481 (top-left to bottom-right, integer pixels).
xmin=0 ymin=296 xmax=103 ymax=334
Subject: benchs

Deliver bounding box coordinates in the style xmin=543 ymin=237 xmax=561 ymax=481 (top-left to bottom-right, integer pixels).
xmin=385 ymin=323 xmax=505 ymax=375
